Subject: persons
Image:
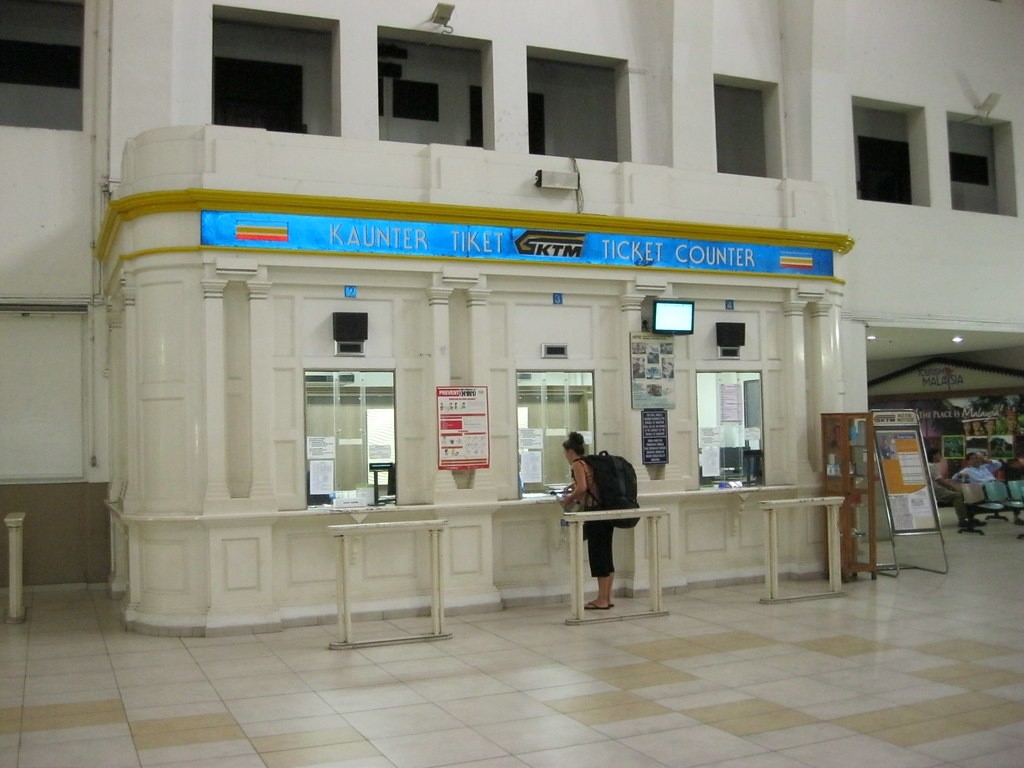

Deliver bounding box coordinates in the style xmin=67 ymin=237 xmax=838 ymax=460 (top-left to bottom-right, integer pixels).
xmin=964 ymin=409 xmax=1022 ymax=440
xmin=559 ymin=432 xmax=617 ymax=609
xmin=928 ymin=448 xmax=1021 ymax=528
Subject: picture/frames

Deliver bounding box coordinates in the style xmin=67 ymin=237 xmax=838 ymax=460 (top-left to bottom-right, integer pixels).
xmin=942 ymin=435 xmax=966 ymax=459
xmin=987 ymin=434 xmax=1015 ymax=459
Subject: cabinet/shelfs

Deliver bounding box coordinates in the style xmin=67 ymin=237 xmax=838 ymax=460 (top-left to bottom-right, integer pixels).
xmin=820 ymin=412 xmax=878 ymax=580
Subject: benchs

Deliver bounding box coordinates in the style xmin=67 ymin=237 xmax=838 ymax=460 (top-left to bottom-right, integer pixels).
xmin=937 ymin=481 xmax=1009 ymax=521
xmin=957 ymin=479 xmax=1024 ymax=536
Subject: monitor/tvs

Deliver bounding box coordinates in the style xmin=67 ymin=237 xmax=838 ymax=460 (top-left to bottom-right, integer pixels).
xmin=652 ymin=300 xmax=695 ymax=335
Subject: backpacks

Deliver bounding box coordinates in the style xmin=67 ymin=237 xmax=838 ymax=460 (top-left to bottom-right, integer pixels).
xmin=572 ymin=451 xmax=640 ymax=529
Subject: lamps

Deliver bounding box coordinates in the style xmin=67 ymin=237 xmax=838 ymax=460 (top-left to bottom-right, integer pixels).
xmin=975 ymin=92 xmax=1002 ymax=115
xmin=430 ymin=2 xmax=456 ymax=25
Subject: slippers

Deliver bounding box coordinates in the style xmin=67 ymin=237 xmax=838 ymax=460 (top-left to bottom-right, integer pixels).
xmin=584 ymin=602 xmax=615 ymax=609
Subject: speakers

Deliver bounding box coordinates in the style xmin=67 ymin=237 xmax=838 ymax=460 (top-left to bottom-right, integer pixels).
xmin=333 ymin=312 xmax=368 ymax=342
xmin=716 ymin=322 xmax=745 ymax=347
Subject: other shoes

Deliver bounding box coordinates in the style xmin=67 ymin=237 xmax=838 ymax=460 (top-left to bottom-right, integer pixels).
xmin=968 ymin=519 xmax=987 ymax=526
xmin=958 ymin=521 xmax=975 ymax=528
